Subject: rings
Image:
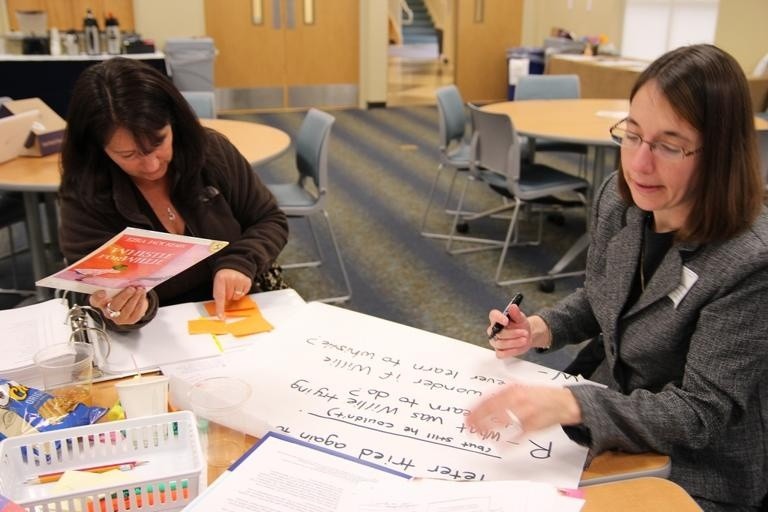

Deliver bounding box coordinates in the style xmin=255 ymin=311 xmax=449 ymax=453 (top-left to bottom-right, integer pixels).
xmin=236 ymin=289 xmax=247 ymax=296
xmin=107 ymin=307 xmax=121 ymax=322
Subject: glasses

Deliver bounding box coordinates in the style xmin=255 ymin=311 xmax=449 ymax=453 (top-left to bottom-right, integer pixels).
xmin=607 ymin=116 xmax=707 ymax=160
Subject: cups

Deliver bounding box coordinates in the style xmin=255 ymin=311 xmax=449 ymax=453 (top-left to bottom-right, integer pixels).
xmin=186 ymin=375 xmax=254 ymax=465
xmin=33 ymin=341 xmax=95 ymax=403
xmin=114 ymin=374 xmax=169 ymax=439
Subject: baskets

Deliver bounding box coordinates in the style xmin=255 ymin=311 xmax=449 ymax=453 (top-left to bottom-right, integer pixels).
xmin=0 ymin=409 xmax=204 ymax=512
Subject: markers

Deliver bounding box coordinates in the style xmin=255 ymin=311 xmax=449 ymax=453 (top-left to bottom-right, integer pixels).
xmin=486 ymin=292 xmax=524 ymax=340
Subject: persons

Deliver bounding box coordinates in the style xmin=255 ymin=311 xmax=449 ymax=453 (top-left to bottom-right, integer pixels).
xmin=55 ymin=58 xmax=288 ymax=332
xmin=466 ymin=45 xmax=767 ymax=512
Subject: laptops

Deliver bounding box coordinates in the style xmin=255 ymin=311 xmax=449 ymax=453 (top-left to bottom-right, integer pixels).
xmin=0 ymin=109 xmax=39 ymax=165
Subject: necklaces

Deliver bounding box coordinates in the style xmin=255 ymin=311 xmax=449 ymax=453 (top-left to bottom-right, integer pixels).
xmin=639 ymin=249 xmax=654 ymax=294
xmin=154 ymin=202 xmax=177 ymax=221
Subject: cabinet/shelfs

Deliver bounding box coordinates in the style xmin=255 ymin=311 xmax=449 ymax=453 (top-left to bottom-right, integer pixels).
xmin=6 ymin=0 xmax=134 ymax=34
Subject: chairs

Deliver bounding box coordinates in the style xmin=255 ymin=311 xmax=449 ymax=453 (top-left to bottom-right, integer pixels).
xmin=0 ymin=97 xmax=44 ymax=295
xmin=264 ymin=107 xmax=353 ymax=305
xmin=503 ymin=74 xmax=588 ymax=213
xmin=420 ymin=84 xmax=526 ymax=248
xmin=165 ymin=37 xmax=215 ymax=117
xmin=445 ymin=100 xmax=594 ymax=287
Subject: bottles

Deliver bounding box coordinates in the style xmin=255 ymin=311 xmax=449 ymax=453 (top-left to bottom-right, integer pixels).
xmin=49 ymin=27 xmax=61 ymax=55
xmin=83 ymin=10 xmax=102 ymax=54
xmin=66 ymin=29 xmax=81 ymax=55
xmin=104 ymin=13 xmax=122 ymax=55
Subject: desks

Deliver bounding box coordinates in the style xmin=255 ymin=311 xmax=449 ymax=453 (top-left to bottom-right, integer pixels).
xmin=42 ymin=370 xmax=674 ymax=487
xmin=0 ymin=118 xmax=293 ymax=301
xmin=482 ymin=98 xmax=767 ymax=294
xmin=580 ymin=476 xmax=707 ymax=512
xmin=543 ymin=52 xmax=767 ymax=114
